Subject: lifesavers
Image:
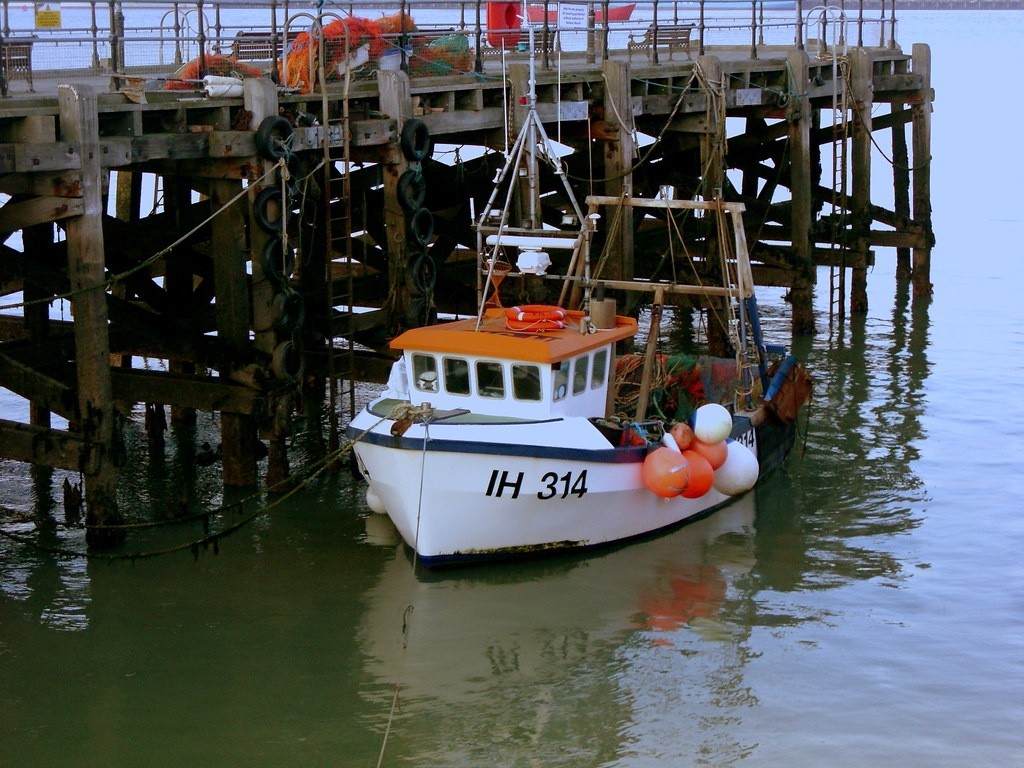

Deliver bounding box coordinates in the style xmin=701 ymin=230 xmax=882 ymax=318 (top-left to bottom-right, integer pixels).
xmin=261 ymin=235 xmax=295 ymax=284
xmin=396 ymin=169 xmax=426 ymax=212
xmin=277 ymin=390 xmax=310 ymax=433
xmin=505 ymin=305 xmax=567 ymax=331
xmin=272 ymin=290 xmax=305 ymax=331
xmin=405 ymin=298 xmax=437 ymax=329
xmin=252 ymin=187 xmax=292 ymax=233
xmin=401 ymin=119 xmax=430 ymax=162
xmin=255 ymin=116 xmax=294 ymax=163
xmin=407 ymin=208 xmax=434 ymax=248
xmin=272 ymin=340 xmax=306 ymax=385
xmin=404 ymin=254 xmax=437 ymax=296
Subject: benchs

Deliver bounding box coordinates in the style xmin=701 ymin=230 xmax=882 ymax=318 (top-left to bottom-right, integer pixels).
xmin=628 ymin=23 xmax=695 ymax=62
xmin=0 ymin=35 xmax=40 ymax=97
xmin=211 ymin=30 xmax=304 ymax=62
xmin=480 ymin=26 xmax=558 ymax=68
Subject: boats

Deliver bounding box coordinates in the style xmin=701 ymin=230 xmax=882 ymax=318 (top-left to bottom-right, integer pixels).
xmin=344 ymin=32 xmax=817 ymax=571
